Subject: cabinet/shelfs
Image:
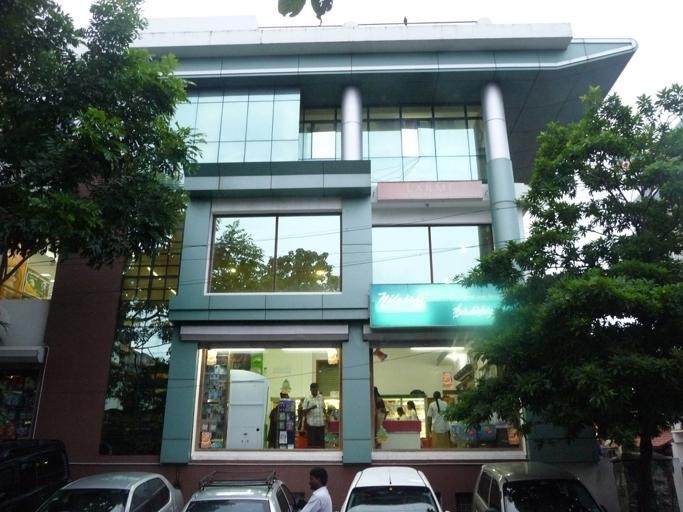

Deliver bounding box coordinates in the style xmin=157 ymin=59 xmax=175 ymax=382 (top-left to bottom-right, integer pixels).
xmin=202 ymin=364 xmax=340 ymax=450
xmin=379 ymin=390 xmax=427 ymax=449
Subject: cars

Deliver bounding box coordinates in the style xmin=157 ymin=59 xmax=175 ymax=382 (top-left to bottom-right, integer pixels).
xmin=35 ymin=470 xmax=184 ymax=512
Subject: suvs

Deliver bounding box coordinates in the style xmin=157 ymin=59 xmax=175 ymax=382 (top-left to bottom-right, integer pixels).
xmin=179 ymin=469 xmax=300 ymax=512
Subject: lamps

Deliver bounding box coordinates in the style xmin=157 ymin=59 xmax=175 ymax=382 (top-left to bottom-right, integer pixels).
xmin=374 ymin=349 xmax=388 ymax=362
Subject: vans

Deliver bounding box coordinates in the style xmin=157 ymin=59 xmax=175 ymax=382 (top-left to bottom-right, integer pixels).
xmin=341 ymin=466 xmax=451 ymax=512
xmin=1 ymin=438 xmax=71 ymax=511
xmin=469 ymin=459 xmax=607 ymax=512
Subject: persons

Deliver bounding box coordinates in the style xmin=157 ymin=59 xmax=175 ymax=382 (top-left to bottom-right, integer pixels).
xmin=426 ymin=391 xmax=451 ymax=449
xmin=297 ymin=395 xmax=304 ymax=430
xmin=299 ymin=381 xmax=330 ymax=448
xmin=406 ymin=401 xmax=418 ymax=419
xmin=373 ymin=386 xmax=387 ymax=448
xmin=296 ymin=465 xmax=332 ymax=511
xmin=397 ymin=406 xmax=407 ymax=421
